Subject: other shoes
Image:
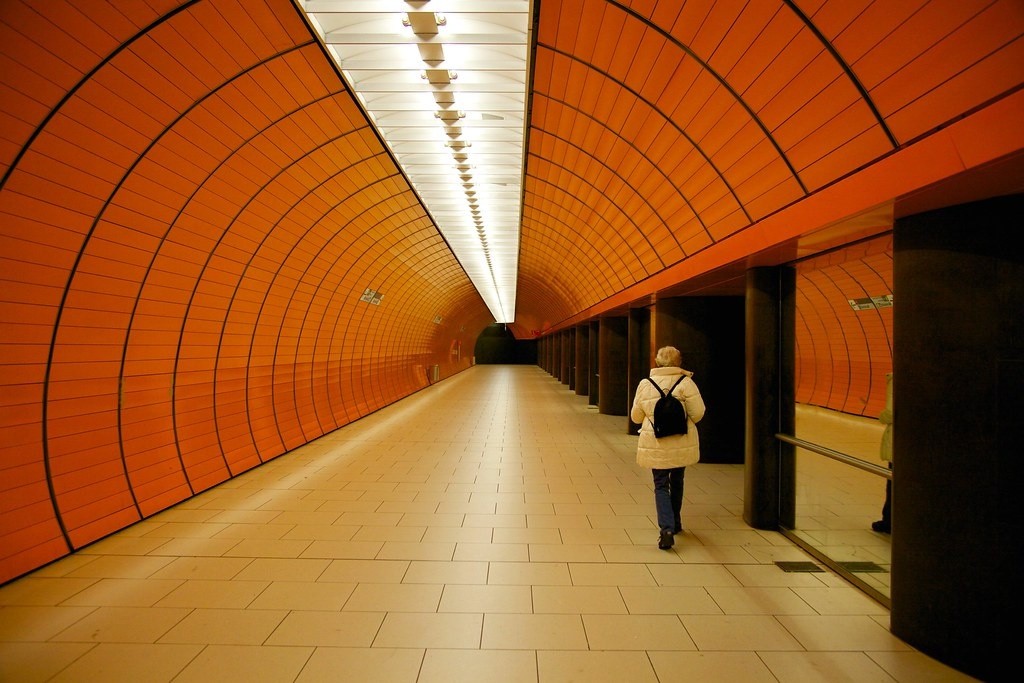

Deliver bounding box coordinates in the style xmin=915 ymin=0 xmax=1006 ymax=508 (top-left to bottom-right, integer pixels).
xmin=872 ymin=521 xmax=891 ymax=534
xmin=659 ymin=532 xmax=674 ymax=549
xmin=672 ymin=527 xmax=681 ymax=534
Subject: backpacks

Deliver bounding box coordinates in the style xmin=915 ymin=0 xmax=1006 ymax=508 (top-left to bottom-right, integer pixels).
xmin=646 ymin=374 xmax=687 ymax=438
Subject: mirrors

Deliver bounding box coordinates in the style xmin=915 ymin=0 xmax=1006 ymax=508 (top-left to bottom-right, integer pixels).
xmin=777 ymin=232 xmax=893 ymax=611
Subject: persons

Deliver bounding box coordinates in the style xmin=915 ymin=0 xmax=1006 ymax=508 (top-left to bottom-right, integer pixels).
xmin=630 ymin=344 xmax=706 ymax=550
xmin=871 ymin=370 xmax=894 ymax=536
